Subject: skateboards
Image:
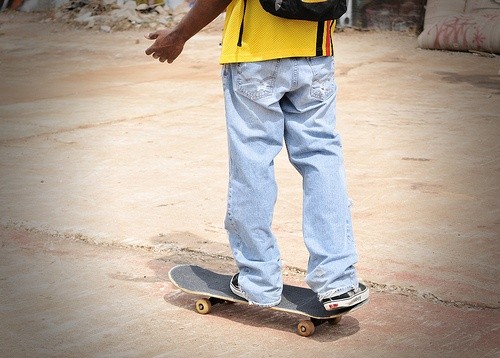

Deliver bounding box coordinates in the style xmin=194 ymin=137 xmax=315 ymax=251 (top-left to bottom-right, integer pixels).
xmin=168 ymin=263 xmax=369 ymax=337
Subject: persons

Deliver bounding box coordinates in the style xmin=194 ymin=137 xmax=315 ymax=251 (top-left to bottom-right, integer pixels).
xmin=143 ymin=0 xmax=370 ymax=311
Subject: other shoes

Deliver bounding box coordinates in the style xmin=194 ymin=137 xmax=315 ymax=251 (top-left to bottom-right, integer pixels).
xmin=230 ymin=272 xmax=251 ymax=299
xmin=323 ymin=282 xmax=369 ymax=310
